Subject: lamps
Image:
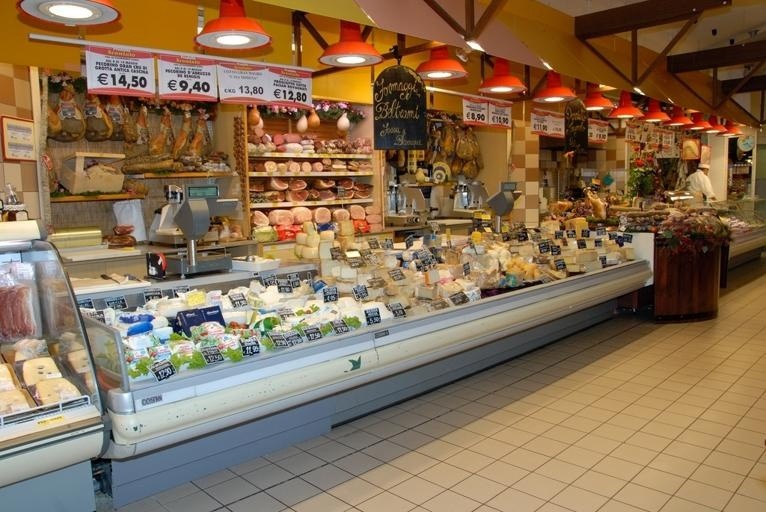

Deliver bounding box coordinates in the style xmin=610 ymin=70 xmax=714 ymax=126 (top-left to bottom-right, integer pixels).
xmin=317 ymin=20 xmax=384 ymax=69
xmin=414 ymin=45 xmax=467 ymax=81
xmin=194 ymin=0 xmax=273 ymax=54
xmin=477 ymin=58 xmax=528 ymax=95
xmin=16 ymin=0 xmax=121 ymax=36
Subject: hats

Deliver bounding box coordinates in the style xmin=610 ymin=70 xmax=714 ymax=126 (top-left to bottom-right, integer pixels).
xmin=698 ymin=164 xmax=710 ymax=169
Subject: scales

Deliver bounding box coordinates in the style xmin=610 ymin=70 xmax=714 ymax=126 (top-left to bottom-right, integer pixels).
xmin=164 ymin=198 xmax=233 ymax=279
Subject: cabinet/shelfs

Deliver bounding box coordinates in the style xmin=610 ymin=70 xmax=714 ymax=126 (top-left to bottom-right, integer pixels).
xmin=1 ymin=241 xmax=109 ymax=512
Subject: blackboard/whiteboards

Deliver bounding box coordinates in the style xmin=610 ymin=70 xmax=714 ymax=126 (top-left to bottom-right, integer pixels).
xmin=564 ymin=100 xmax=590 ymax=159
xmin=373 ymin=63 xmax=427 ymax=150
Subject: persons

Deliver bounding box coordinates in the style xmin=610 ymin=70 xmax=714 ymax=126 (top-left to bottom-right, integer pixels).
xmin=675 ymin=164 xmax=717 ymax=201
